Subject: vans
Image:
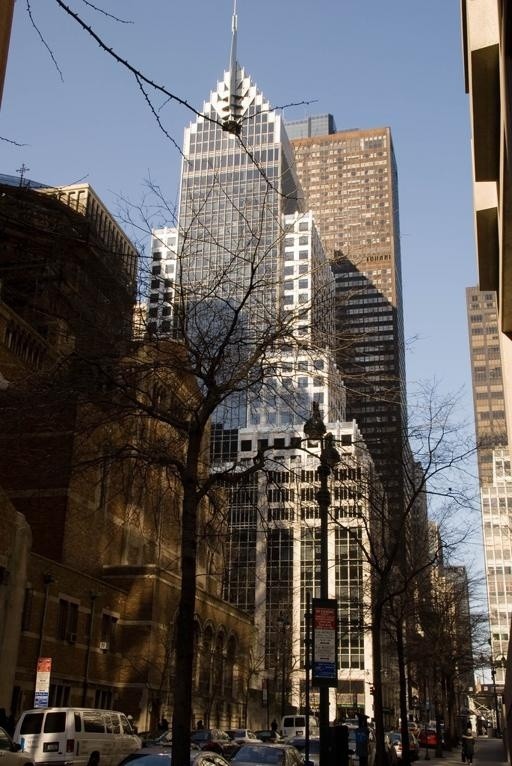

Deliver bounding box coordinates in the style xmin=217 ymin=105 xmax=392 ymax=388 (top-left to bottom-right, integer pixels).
xmin=280 ymin=715 xmax=319 ymax=738
xmin=331 ymin=724 xmax=378 ymax=766
xmin=13 ymin=708 xmax=142 ymax=766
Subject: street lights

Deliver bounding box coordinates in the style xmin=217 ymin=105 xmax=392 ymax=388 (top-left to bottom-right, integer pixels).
xmin=304 ymin=401 xmax=343 ymax=766
xmin=479 ymin=652 xmax=506 ymax=740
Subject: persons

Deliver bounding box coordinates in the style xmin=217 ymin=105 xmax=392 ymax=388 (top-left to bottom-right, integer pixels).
xmin=197 ymin=719 xmax=204 ymax=728
xmin=271 ymin=718 xmax=278 ymax=731
xmin=462 ymin=728 xmax=475 ymax=764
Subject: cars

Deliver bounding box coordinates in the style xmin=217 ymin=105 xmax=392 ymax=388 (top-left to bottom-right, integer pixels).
xmin=255 ymin=729 xmax=281 ymax=741
xmin=122 ymin=750 xmax=231 ymax=766
xmin=390 ymin=732 xmax=420 ymax=757
xmin=227 ymin=743 xmax=315 ymax=766
xmin=192 ymin=728 xmax=229 ymax=745
xmin=415 ymin=728 xmax=437 ymax=747
xmin=283 ymin=735 xmax=355 ymax=766
xmin=1 ymin=727 xmax=35 ymax=765
xmin=224 ymin=729 xmax=264 ymax=742
xmin=383 ymin=713 xmax=444 ymax=736
